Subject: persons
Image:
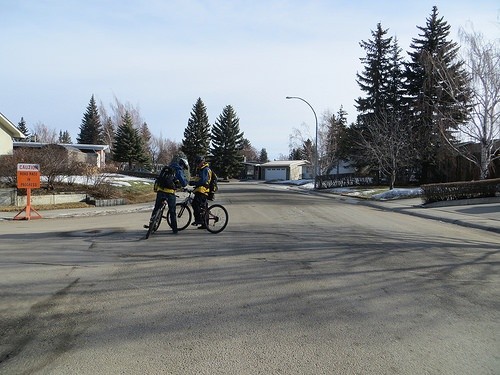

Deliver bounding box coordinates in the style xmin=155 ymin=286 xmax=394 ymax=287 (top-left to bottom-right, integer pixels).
xmin=150 ymin=158 xmax=188 ymax=234
xmin=188 ymin=154 xmax=217 ymax=230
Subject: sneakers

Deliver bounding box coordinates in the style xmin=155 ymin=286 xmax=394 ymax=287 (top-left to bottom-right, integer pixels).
xmin=173 ymin=230 xmax=178 ymax=233
xmin=192 ymin=222 xmax=207 ymax=229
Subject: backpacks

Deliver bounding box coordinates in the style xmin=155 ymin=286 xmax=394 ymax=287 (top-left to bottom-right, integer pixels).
xmin=160 ymin=166 xmax=178 ymax=190
xmin=203 ymin=171 xmax=218 ymax=192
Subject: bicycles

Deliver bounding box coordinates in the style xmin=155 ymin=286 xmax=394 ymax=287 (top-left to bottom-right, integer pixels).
xmin=143 ymin=190 xmax=179 ymax=239
xmin=166 ymin=184 xmax=228 ymax=234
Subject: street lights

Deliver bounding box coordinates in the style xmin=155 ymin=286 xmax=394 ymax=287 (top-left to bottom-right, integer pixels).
xmin=286 ymin=96 xmax=318 ymax=188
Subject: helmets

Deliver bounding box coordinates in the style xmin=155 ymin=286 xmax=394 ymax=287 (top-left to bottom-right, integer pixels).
xmin=196 ymin=156 xmax=205 ymax=164
xmin=180 ymin=158 xmax=189 ymax=170
